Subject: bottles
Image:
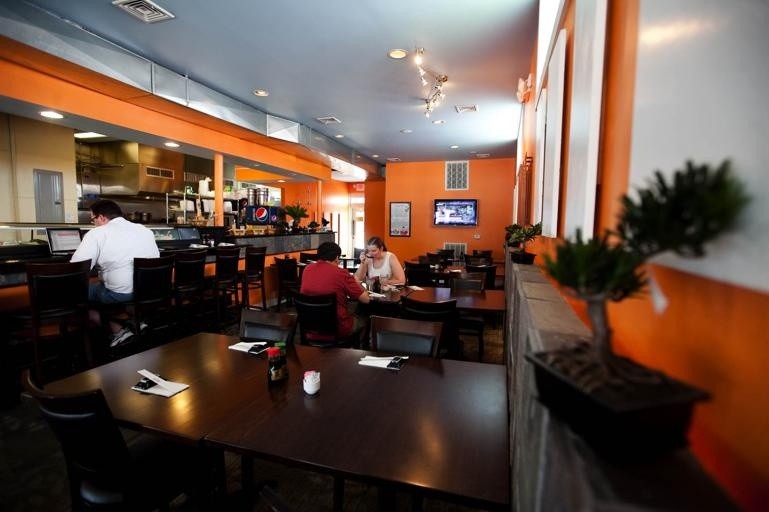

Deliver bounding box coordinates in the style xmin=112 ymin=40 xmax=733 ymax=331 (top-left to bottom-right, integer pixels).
xmin=268 ymin=341 xmax=288 ymax=385
xmin=373 ymin=276 xmax=380 ymax=294
xmin=202 ymin=233 xmax=211 ymax=246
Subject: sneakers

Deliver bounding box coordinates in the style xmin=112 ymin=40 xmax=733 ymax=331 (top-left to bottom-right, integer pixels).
xmin=130 ymin=322 xmax=147 ymax=334
xmin=110 ymin=327 xmax=134 ymax=348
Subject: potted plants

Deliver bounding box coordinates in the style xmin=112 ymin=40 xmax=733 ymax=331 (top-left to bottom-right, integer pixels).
xmin=309 ymin=219 xmax=320 ymax=233
xmin=284 ymin=201 xmax=309 ymax=233
xmin=513 ymin=158 xmax=757 ymax=456
xmin=510 ymin=221 xmax=542 ymax=266
xmin=320 ymin=216 xmax=330 ymax=233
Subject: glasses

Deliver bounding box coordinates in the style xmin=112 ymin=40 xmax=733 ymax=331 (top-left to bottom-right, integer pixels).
xmin=89 ymin=216 xmax=100 ymax=224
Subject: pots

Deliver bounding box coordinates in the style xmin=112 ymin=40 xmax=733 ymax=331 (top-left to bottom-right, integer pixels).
xmin=135 ymin=211 xmax=152 ymax=224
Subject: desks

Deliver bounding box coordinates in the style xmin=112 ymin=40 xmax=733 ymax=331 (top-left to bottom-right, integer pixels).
xmin=337 ymin=254 xmax=356 ymax=268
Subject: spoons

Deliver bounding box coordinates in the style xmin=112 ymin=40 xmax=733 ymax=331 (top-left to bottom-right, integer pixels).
xmin=364 ymin=253 xmax=374 ymax=258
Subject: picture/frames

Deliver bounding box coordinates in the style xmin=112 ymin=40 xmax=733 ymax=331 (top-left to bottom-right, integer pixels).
xmin=390 ymin=202 xmax=411 ymax=238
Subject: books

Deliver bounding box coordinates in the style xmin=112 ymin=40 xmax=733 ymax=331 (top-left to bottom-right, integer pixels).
xmin=352 ymin=236 xmax=406 ymax=334
xmin=358 ymin=355 xmax=409 ymax=371
xmin=227 ymin=342 xmax=270 ymax=354
xmin=130 ymin=369 xmax=190 ymax=398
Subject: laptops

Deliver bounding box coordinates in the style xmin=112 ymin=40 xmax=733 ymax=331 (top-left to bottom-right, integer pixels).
xmin=45 ymin=228 xmax=82 ymax=255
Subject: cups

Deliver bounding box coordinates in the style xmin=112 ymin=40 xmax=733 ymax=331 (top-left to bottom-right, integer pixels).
xmin=303 ymin=379 xmax=320 ymax=395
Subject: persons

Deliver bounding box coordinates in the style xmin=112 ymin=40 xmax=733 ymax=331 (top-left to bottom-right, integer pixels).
xmin=70 ymin=199 xmax=160 ymax=347
xmin=300 ymin=242 xmax=369 ymax=350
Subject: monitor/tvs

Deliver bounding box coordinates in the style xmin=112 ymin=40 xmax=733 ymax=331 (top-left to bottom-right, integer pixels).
xmin=433 ymin=199 xmax=477 ymax=226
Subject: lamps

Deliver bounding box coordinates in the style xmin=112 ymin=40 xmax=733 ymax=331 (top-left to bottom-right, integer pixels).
xmin=414 ymin=42 xmax=451 ymax=121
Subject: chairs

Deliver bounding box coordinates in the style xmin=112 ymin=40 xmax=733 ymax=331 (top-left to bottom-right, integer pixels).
xmin=273 ymin=256 xmax=300 ymax=304
xmin=240 ymin=247 xmax=271 ymax=312
xmin=449 ymin=270 xmax=486 ymax=291
xmin=402 ymin=261 xmax=434 ymax=285
xmin=369 ymin=314 xmax=444 ymax=360
xmin=239 ymin=302 xmax=297 ymax=344
xmin=207 ymin=248 xmax=239 ymax=308
xmin=294 ymin=289 xmax=360 ymax=350
xmin=21 ymin=374 xmax=230 ymax=507
xmin=23 ymin=256 xmax=94 ymax=357
xmin=299 ymin=252 xmax=323 ymax=262
xmin=465 ymin=263 xmax=498 ymax=286
xmin=177 ymin=251 xmax=207 ymax=324
xmin=103 ymin=253 xmax=177 ymax=339
